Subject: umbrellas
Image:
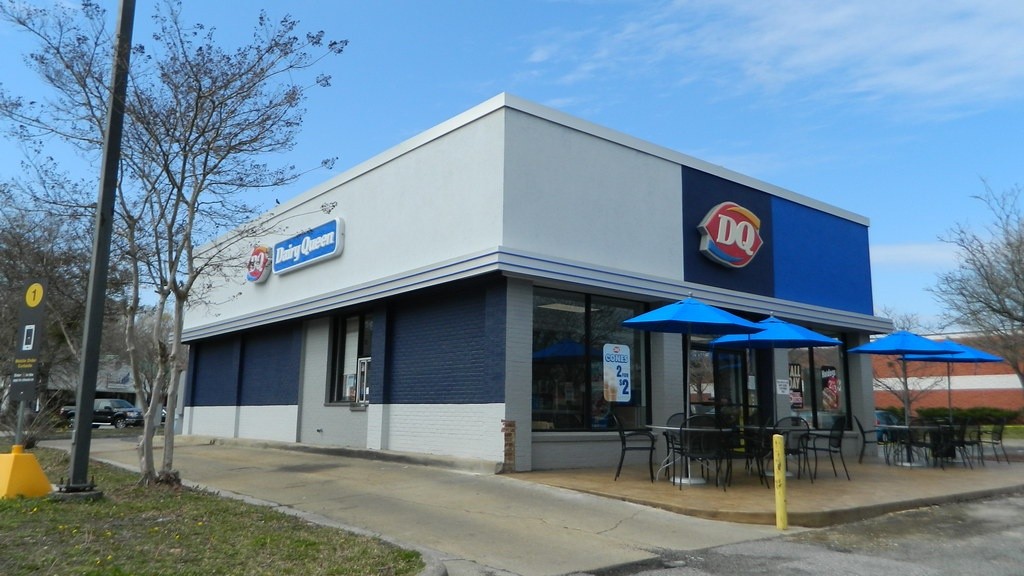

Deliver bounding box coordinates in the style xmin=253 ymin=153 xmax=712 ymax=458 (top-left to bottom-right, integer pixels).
xmin=532 ymin=338 xmax=604 ymax=359
xmin=711 ymin=311 xmax=844 ymax=427
xmin=898 ymin=336 xmax=1005 ymax=424
xmin=846 ymin=327 xmax=966 ymax=460
xmin=621 ymin=292 xmax=768 ymax=478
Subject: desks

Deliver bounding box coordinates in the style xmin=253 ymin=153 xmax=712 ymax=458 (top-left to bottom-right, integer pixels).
xmin=872 ymin=424 xmax=942 ymax=467
xmin=736 ymin=425 xmax=815 ymax=477
xmin=644 ymin=424 xmax=732 ymax=485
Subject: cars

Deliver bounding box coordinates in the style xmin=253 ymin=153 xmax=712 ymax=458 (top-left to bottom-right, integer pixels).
xmin=875 ymin=410 xmax=907 ymax=443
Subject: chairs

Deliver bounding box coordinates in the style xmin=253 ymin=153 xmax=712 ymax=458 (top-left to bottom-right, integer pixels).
xmin=853 ymin=415 xmax=890 ymax=464
xmin=797 ymin=415 xmax=850 ymax=480
xmin=612 ymin=413 xmax=656 ymax=481
xmin=878 ymin=415 xmax=1012 ymax=469
xmin=662 ymin=413 xmax=813 ymax=492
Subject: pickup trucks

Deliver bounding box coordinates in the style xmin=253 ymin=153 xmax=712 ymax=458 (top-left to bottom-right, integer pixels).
xmin=60 ymin=398 xmax=144 ymax=429
